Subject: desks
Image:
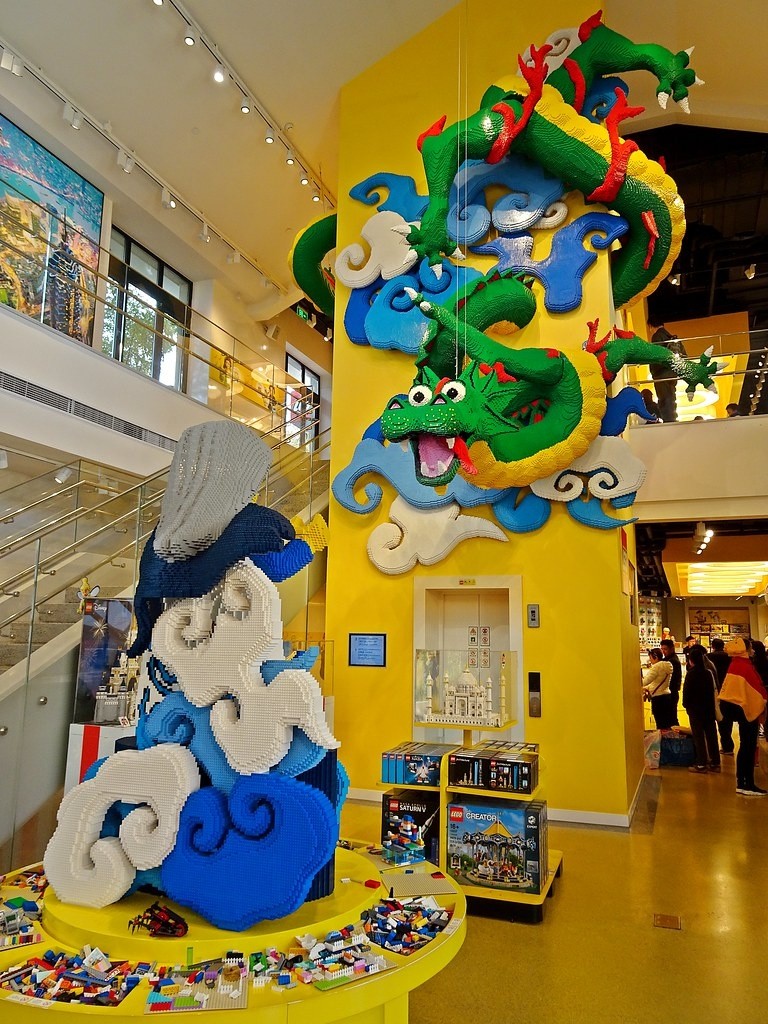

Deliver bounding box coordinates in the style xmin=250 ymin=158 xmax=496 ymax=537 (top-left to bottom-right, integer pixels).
xmin=0 ymin=837 xmax=467 ymax=1024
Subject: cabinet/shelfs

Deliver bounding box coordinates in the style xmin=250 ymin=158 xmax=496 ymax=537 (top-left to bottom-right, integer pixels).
xmin=375 ymin=722 xmax=564 ymax=925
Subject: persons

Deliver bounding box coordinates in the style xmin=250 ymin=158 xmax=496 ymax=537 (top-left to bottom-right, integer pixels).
xmin=642 ymin=639 xmax=681 ymax=730
xmin=682 ymin=636 xmax=768 ymax=796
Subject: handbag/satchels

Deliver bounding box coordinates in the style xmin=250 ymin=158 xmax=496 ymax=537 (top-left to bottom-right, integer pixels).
xmin=714 ymin=688 xmax=724 ymax=722
xmin=643 ymin=689 xmax=651 ymax=701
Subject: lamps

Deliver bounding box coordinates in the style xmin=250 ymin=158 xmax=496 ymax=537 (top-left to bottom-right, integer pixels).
xmin=745 ymin=270 xmax=754 ymax=279
xmin=311 ymin=188 xmax=320 ymax=203
xmin=667 ymin=275 xmax=677 ymax=285
xmin=54 ymin=468 xmax=72 ymax=484
xmin=696 ymin=523 xmax=714 ymax=537
xmin=299 ymin=171 xmax=309 ymax=186
xmin=234 ymin=253 xmax=240 ymax=264
xmin=240 ymin=96 xmax=251 ymax=115
xmin=323 ymin=329 xmax=332 ymax=341
xmin=161 ymin=190 xmax=176 ymax=210
xmin=70 ymin=113 xmax=84 ymax=131
xmin=265 ymin=128 xmax=275 ymax=145
xmin=0 ymin=450 xmax=8 ymax=470
xmin=306 ymin=315 xmax=316 ymax=329
xmin=286 ymin=150 xmax=295 ymax=166
xmin=748 ymin=356 xmax=768 ymax=416
xmin=122 ymin=159 xmax=134 ymax=175
xmin=693 ymin=531 xmax=711 ymax=543
xmin=184 ymin=29 xmax=196 ymax=48
xmin=11 ymin=58 xmax=25 ymax=79
xmin=214 ymin=64 xmax=224 ymax=83
xmin=198 ymin=226 xmax=211 ymax=243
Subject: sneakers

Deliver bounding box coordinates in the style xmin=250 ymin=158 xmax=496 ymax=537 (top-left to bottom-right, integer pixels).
xmin=708 ymin=763 xmax=721 ymax=772
xmin=735 ymin=780 xmax=768 ymax=796
xmin=688 ymin=764 xmax=709 ymax=774
xmin=759 ymin=726 xmax=764 ymax=738
xmin=720 ymin=748 xmax=733 ymax=755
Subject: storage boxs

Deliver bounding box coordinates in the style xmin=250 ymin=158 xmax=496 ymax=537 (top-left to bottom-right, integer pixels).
xmin=382 ymin=741 xmax=412 ymax=783
xmin=396 ymin=743 xmax=426 ymax=784
xmin=404 ymin=745 xmax=438 ymax=785
xmin=691 ymin=624 xmax=701 ymax=632
xmin=700 ymin=636 xmax=709 ymax=648
xmin=691 ymin=634 xmax=700 ymax=641
xmin=453 ymin=794 xmax=549 ymax=888
xmin=448 ymin=740 xmax=540 ymax=794
xmin=723 ymin=625 xmax=729 ymax=632
xmin=728 ymin=624 xmax=749 ymax=633
xmin=701 ymin=625 xmax=711 ymax=632
xmin=711 ymin=625 xmax=723 ymax=632
xmin=387 ymin=795 xmax=439 ymax=866
xmin=381 ymin=788 xmax=406 ymax=846
xmin=446 ymin=801 xmax=543 ymax=896
xmin=423 ymin=745 xmax=458 ymax=788
xmin=388 ymin=742 xmax=419 ymax=784
